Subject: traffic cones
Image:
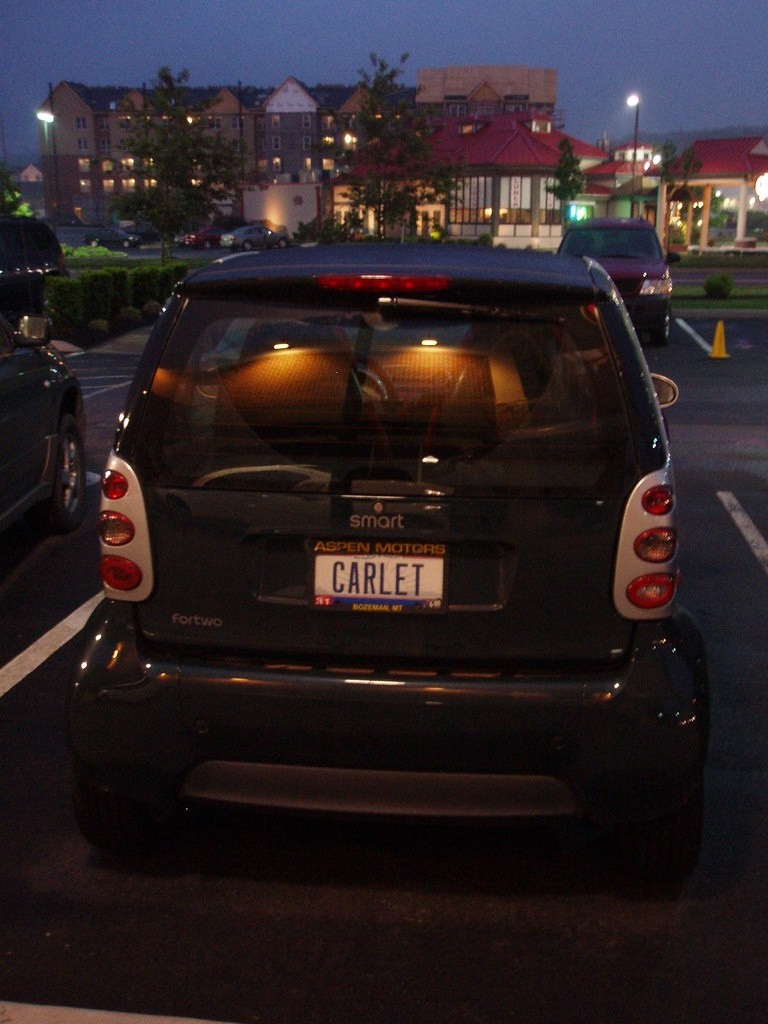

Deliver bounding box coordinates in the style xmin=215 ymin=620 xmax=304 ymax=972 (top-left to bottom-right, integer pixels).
xmin=707 ymin=320 xmax=731 ymax=359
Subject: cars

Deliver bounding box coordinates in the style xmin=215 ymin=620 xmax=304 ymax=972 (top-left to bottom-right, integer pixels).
xmin=0 ymin=312 xmax=86 ymax=534
xmin=175 ymin=224 xmax=293 ymax=252
xmin=84 ymin=223 xmax=163 ymax=250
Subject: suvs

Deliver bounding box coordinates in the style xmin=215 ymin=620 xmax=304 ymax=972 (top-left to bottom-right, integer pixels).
xmin=556 ymin=216 xmax=681 ymax=346
xmin=63 ymin=246 xmax=714 ymax=884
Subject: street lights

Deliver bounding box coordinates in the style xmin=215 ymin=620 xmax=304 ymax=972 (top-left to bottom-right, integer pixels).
xmin=626 ymin=90 xmax=639 ymax=217
xmin=37 ymin=112 xmax=55 ymax=232
